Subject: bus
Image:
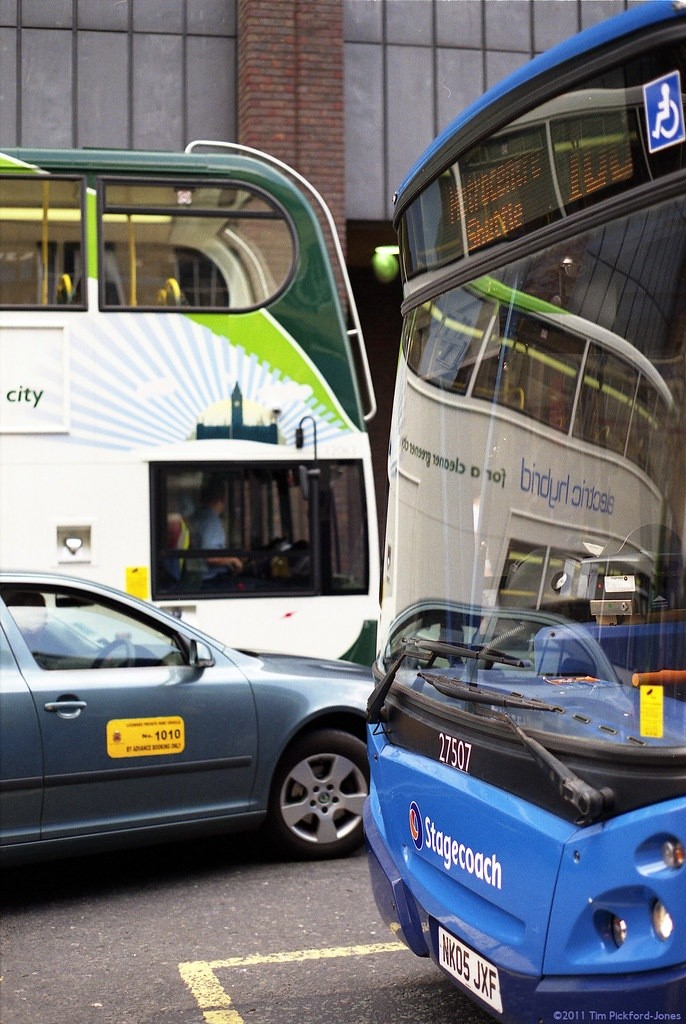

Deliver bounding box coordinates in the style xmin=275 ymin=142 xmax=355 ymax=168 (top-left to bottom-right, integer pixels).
xmin=0 ymin=138 xmax=377 ymax=664
xmin=353 ymin=0 xmax=686 ymax=1024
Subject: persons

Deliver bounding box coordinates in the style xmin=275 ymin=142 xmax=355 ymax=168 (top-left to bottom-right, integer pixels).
xmin=7 ymin=591 xmax=48 ymax=674
xmin=190 ymin=481 xmax=243 ymax=590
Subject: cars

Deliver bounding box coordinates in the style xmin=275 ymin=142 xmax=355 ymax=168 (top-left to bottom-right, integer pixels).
xmin=0 ymin=570 xmax=376 ymax=866
